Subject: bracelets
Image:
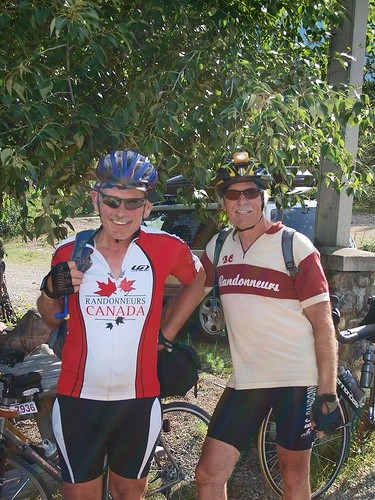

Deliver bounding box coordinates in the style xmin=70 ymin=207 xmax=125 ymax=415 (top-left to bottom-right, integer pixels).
xmin=157 ymin=329 xmax=173 ymax=348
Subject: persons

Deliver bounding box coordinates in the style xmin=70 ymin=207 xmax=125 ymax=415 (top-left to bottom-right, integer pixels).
xmin=160 ymin=157 xmax=338 ymax=500
xmin=37 ymin=151 xmax=207 ymax=500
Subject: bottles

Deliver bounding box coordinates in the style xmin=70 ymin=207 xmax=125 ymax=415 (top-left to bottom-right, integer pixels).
xmin=43 ymin=439 xmax=62 ymax=475
xmin=337 ymin=366 xmax=365 ymax=400
xmin=360 ymin=346 xmax=375 ymax=388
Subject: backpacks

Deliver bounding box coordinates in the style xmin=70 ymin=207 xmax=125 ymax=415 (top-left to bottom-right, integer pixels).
xmin=49 ymin=230 xmax=201 ymax=397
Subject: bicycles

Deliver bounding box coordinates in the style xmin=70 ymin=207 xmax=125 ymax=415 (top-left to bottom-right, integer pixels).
xmin=0 ymin=370 xmax=216 ymax=500
xmin=258 ymin=296 xmax=374 ymax=499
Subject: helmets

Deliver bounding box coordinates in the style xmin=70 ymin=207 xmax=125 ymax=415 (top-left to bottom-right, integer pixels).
xmin=212 ymin=152 xmax=272 ymax=193
xmin=91 ymin=150 xmax=158 ymax=193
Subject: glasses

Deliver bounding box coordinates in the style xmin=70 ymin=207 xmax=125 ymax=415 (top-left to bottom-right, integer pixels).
xmin=222 ymin=188 xmax=262 ymax=201
xmin=100 ymin=190 xmax=149 ymax=210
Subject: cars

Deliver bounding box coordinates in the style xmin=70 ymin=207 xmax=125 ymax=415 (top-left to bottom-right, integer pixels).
xmin=144 ymin=165 xmax=319 ymax=341
